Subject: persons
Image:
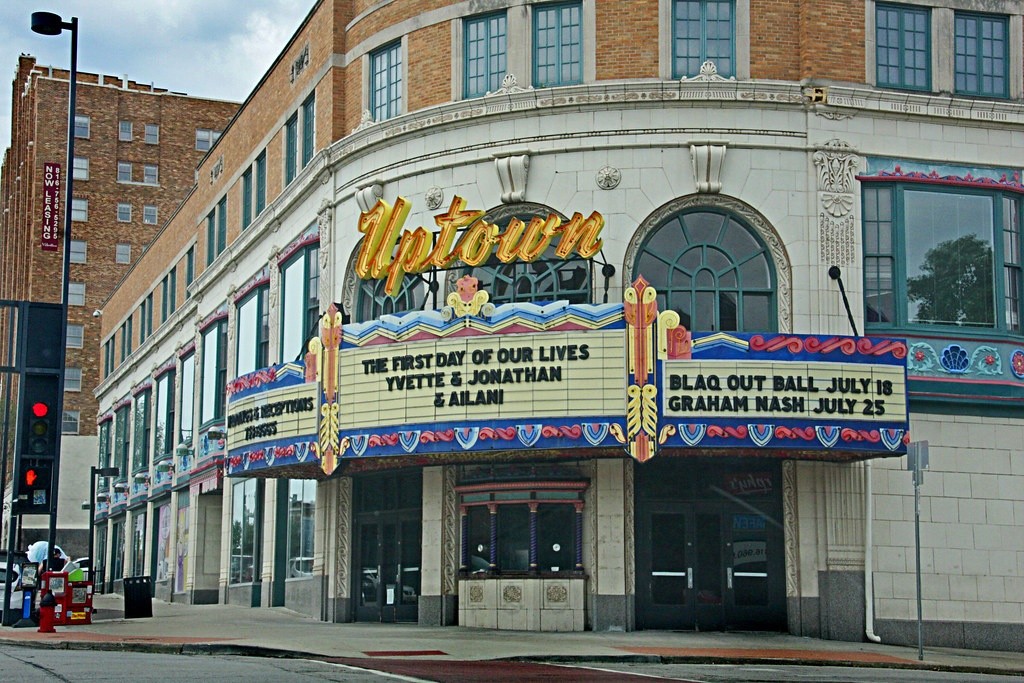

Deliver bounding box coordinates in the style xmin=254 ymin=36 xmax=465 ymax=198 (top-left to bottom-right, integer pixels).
xmin=34 ymin=548 xmax=67 ymax=578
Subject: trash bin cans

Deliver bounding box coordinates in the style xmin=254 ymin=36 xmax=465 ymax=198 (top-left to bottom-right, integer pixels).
xmin=66 ymin=580 xmax=94 ymax=625
xmin=40 ymin=571 xmax=69 ymax=626
xmin=123 ymin=575 xmax=153 ymax=619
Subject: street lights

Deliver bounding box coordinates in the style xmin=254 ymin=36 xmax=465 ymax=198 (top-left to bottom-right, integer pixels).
xmin=88 ymin=466 xmax=119 ymax=613
xmin=31 ymin=12 xmax=78 ymax=570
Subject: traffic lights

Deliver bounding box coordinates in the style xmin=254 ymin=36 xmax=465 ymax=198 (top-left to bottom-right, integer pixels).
xmin=11 ymin=300 xmax=68 ymax=515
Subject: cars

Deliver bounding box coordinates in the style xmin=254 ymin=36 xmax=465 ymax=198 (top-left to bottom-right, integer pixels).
xmin=289 ymin=556 xmax=314 ymax=578
xmin=0 ymin=562 xmax=23 ymax=624
xmin=361 ymin=566 xmax=419 ymax=602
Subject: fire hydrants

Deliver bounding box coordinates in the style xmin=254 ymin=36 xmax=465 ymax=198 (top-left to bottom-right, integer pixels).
xmin=38 ymin=590 xmax=59 ymax=632
